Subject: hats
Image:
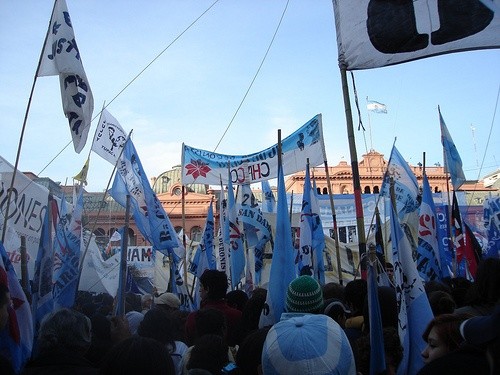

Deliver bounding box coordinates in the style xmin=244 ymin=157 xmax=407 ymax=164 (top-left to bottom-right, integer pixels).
xmin=261 ymin=315 xmax=356 ymax=375
xmin=460 ymin=306 xmax=500 ymax=346
xmin=323 ymin=282 xmax=343 ymax=298
xmin=286 ymin=275 xmax=323 ymax=313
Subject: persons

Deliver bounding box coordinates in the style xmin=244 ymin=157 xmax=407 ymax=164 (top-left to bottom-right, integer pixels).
xmin=0 ymin=270 xmax=499 ymax=375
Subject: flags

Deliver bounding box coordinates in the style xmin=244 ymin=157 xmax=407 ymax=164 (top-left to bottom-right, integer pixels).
xmin=388 ymin=183 xmax=438 ymax=374
xmin=36 ymin=0 xmax=92 ymax=152
xmin=331 ymin=0 xmax=500 ymax=67
xmin=0 ymin=101 xmax=500 ymax=326
xmin=0 ymin=232 xmax=34 ymax=375
